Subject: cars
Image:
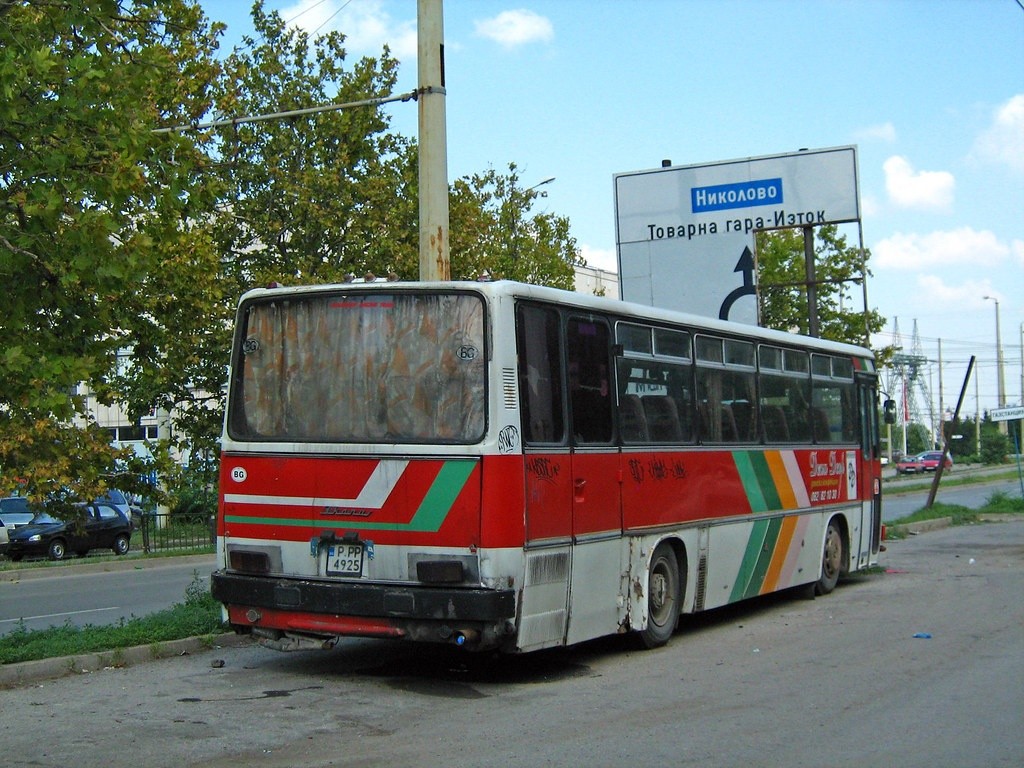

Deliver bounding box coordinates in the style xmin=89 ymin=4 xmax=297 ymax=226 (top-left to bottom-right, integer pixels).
xmin=7 ymin=503 xmax=132 ymax=561
xmin=916 ymin=451 xmax=952 ymax=473
xmin=892 ymin=450 xmax=904 ymax=461
xmin=88 ymin=489 xmax=132 ymax=526
xmin=896 ymin=455 xmax=921 ymax=475
xmin=0 ymin=497 xmax=38 ymax=545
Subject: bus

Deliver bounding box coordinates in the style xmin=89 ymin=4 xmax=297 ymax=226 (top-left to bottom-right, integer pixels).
xmin=210 ymin=278 xmax=897 ymax=652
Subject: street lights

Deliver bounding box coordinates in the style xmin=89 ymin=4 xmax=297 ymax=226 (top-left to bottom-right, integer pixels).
xmin=499 ymin=178 xmax=555 ymax=228
xmin=983 ymin=295 xmax=1008 ymax=435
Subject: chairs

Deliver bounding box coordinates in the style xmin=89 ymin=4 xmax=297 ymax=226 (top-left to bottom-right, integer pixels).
xmin=614 ymin=394 xmax=833 ymax=443
